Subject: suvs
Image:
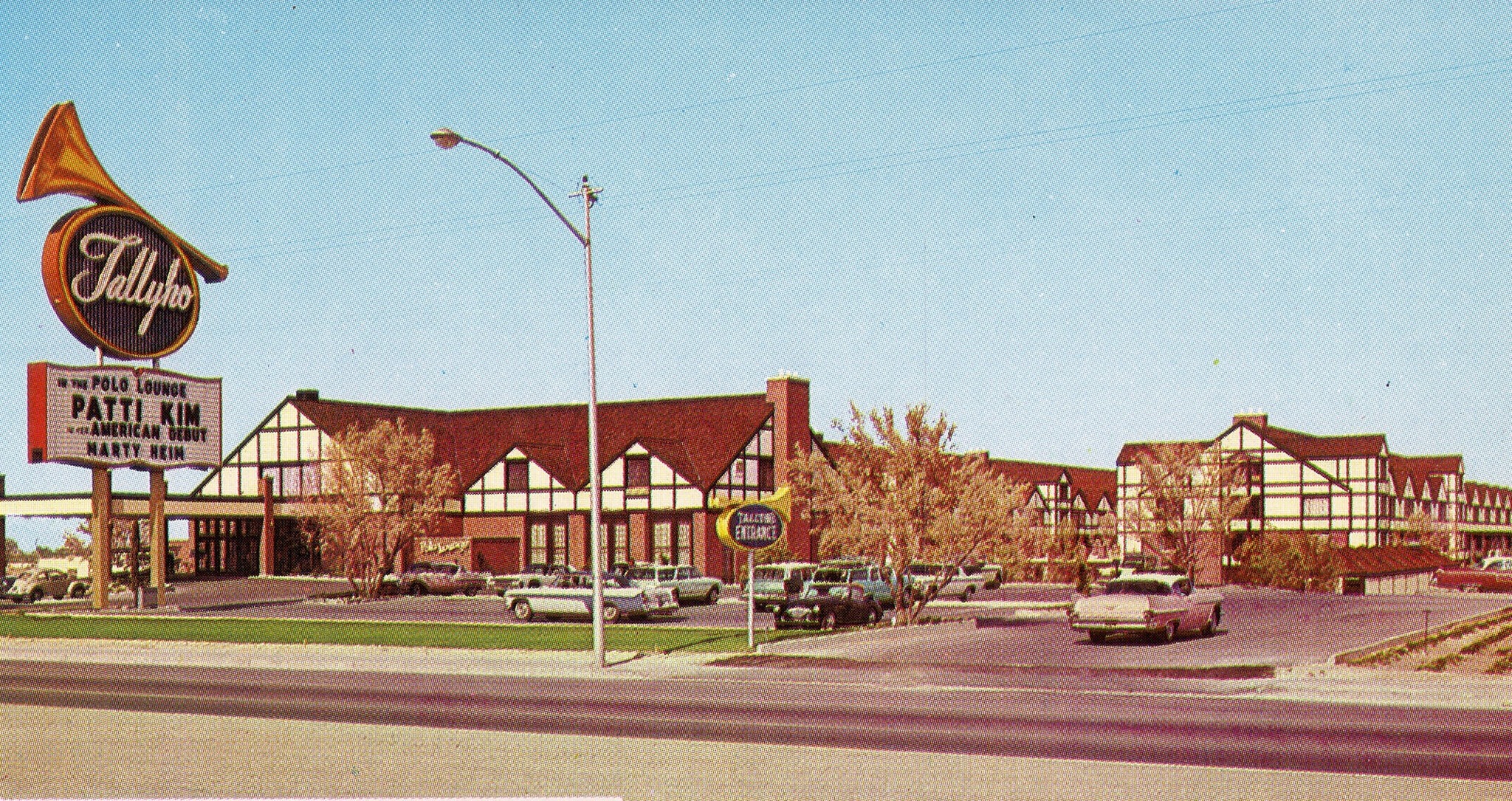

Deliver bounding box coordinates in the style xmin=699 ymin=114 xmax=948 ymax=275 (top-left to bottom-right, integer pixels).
xmin=743 ymin=559 xmax=815 ymax=611
xmin=803 ymin=557 xmax=895 ymax=611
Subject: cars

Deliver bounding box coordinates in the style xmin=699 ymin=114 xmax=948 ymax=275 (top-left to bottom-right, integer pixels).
xmin=1069 ymin=570 xmax=1227 ymax=642
xmin=493 ymin=559 xmax=575 ymax=598
xmin=897 ymin=562 xmax=981 ymax=603
xmin=502 ymin=572 xmax=679 ymax=615
xmin=965 ymin=558 xmax=1008 ymax=591
xmin=398 ymin=564 xmax=487 ymax=596
xmin=628 ymin=562 xmax=725 ymax=606
xmin=1430 ymin=553 xmax=1511 ymax=592
xmin=779 ymin=582 xmax=881 ymax=631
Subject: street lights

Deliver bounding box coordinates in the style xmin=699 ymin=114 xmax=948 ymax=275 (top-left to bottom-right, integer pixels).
xmin=434 ymin=122 xmax=609 ymax=661
